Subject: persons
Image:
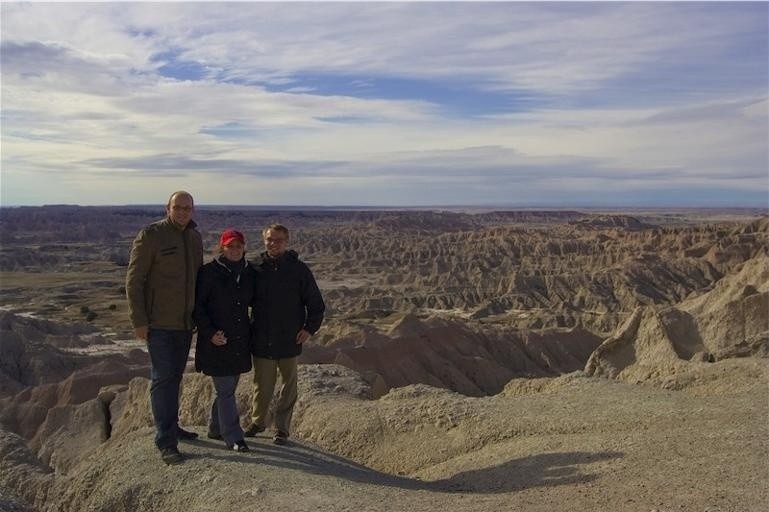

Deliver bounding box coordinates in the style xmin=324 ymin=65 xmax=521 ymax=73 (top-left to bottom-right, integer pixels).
xmin=125 ymin=188 xmax=206 ymax=464
xmin=244 ymin=223 xmax=326 ymax=444
xmin=194 ymin=229 xmax=256 ymax=453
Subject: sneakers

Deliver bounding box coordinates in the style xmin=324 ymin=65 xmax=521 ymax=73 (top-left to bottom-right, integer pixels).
xmin=177 ymin=426 xmax=199 ymax=438
xmin=161 ymin=446 xmax=182 ymax=464
xmin=244 ymin=423 xmax=265 ymax=436
xmin=273 ymin=431 xmax=287 ymax=444
xmin=229 ymin=441 xmax=249 ymax=452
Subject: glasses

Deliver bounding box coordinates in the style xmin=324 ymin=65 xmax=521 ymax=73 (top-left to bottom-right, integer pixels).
xmin=170 ymin=205 xmax=194 ymax=212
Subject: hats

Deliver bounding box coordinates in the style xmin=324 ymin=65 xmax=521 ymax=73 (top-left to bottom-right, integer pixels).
xmin=220 ymin=231 xmax=244 ymax=247
